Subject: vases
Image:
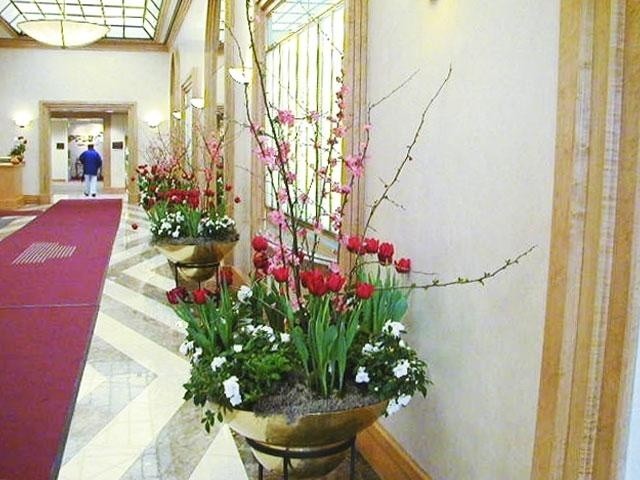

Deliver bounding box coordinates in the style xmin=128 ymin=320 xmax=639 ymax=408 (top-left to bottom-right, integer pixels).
xmin=157 ymin=241 xmax=241 ymax=281
xmin=208 ymin=396 xmax=393 ymax=480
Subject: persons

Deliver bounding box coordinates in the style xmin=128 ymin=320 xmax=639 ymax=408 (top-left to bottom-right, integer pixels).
xmin=80 ymin=145 xmax=102 ymax=197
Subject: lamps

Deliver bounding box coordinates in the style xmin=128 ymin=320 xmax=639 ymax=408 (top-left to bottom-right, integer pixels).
xmin=16 ymin=17 xmax=113 ymax=52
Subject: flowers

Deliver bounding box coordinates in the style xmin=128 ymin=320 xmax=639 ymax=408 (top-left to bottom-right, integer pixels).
xmin=166 ymin=57 xmax=478 ymax=429
xmin=132 ymin=112 xmax=246 ymax=240
